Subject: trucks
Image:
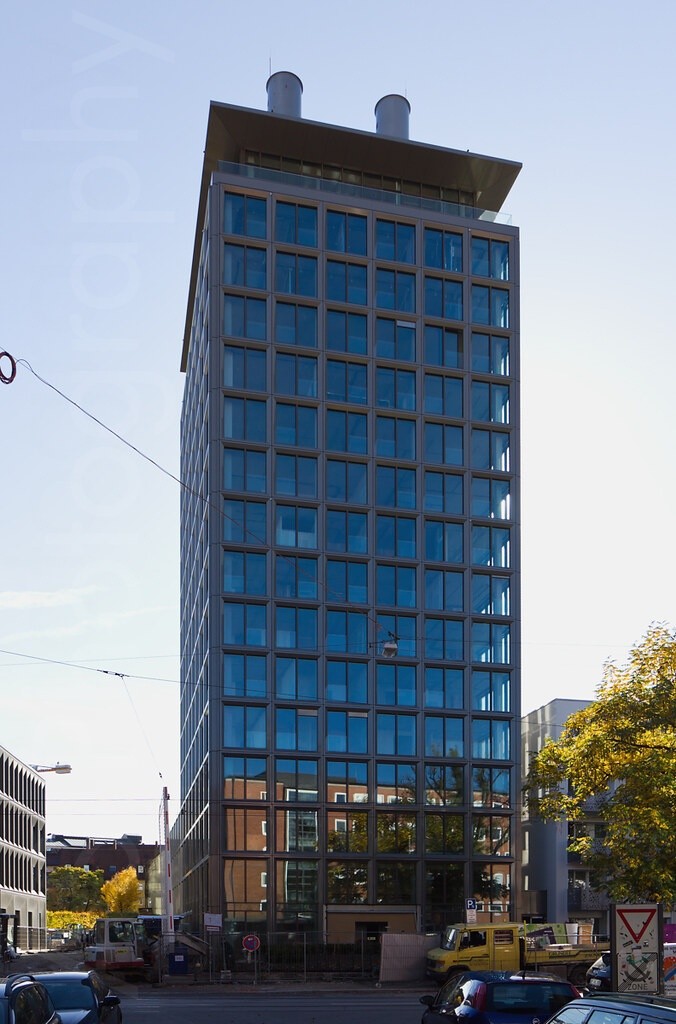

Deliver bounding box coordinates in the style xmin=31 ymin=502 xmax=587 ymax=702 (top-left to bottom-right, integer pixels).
xmin=424 ymin=920 xmax=610 ymax=989
xmin=586 ymin=941 xmax=676 ymax=999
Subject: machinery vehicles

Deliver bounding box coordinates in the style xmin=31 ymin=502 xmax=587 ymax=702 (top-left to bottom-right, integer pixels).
xmin=82 ymin=916 xmax=217 ymax=988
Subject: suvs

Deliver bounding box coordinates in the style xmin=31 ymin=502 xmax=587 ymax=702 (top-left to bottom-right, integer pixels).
xmin=0 ymin=972 xmax=64 ymax=1024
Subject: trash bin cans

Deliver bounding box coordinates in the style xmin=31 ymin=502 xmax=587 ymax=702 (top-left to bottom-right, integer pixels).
xmin=167 ymin=952 xmax=184 ymax=975
xmin=175 ymin=948 xmax=188 ymax=974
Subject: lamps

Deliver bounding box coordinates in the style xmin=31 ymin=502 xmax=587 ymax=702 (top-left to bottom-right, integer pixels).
xmin=380 ymin=639 xmax=400 ymax=660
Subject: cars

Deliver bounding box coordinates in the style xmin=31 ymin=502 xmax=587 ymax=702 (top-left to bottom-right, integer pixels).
xmin=542 ymin=990 xmax=676 ymax=1024
xmin=18 ymin=969 xmax=123 ymax=1024
xmin=418 ymin=969 xmax=591 ymax=1024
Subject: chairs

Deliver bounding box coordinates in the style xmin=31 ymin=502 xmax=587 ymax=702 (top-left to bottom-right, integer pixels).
xmin=109 ymin=927 xmax=119 ymax=942
xmin=526 ymin=986 xmax=543 ymax=1003
